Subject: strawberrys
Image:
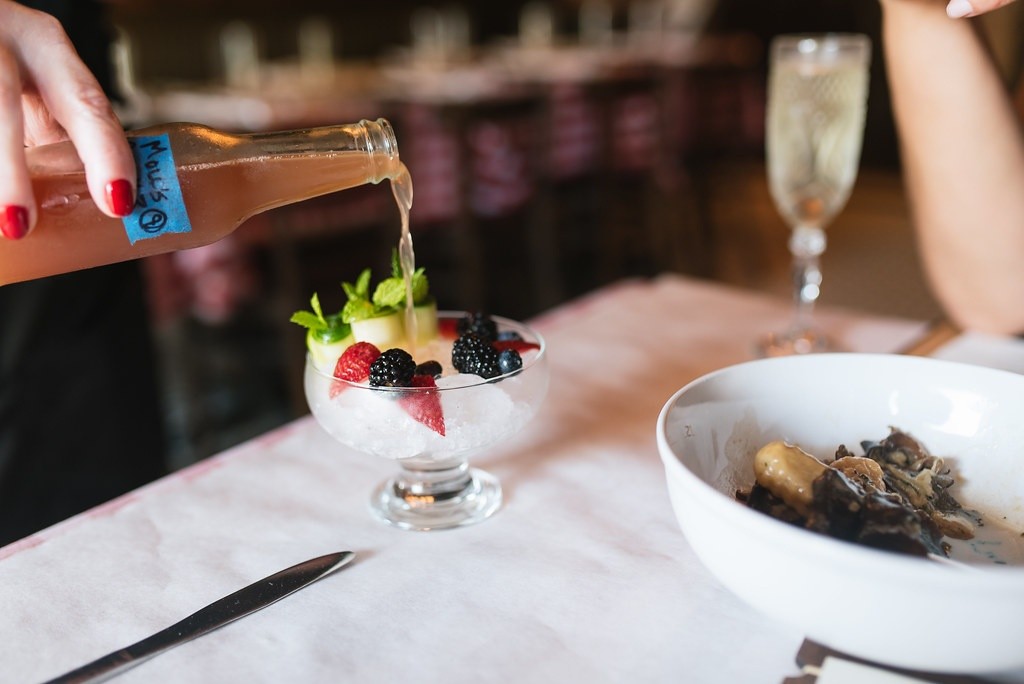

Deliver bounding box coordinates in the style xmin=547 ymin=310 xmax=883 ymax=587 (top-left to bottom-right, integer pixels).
xmin=330 ymin=342 xmax=380 ymax=400
xmin=399 ymin=376 xmax=445 ymax=438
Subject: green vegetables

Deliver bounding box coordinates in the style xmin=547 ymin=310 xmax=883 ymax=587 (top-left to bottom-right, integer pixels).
xmin=291 ymin=248 xmax=424 ymax=331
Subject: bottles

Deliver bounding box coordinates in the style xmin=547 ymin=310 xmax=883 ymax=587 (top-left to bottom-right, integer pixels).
xmin=1 ymin=118 xmax=401 ymax=289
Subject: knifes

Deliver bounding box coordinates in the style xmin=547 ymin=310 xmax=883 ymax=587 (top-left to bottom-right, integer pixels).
xmin=42 ymin=550 xmax=354 ymax=684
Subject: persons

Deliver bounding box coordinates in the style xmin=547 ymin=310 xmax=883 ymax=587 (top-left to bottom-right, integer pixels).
xmin=0 ymin=0 xmax=138 ymax=240
xmin=878 ymin=0 xmax=1024 ymax=339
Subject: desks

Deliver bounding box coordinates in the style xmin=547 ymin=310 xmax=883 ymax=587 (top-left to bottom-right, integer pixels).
xmin=0 ymin=273 xmax=1024 ymax=684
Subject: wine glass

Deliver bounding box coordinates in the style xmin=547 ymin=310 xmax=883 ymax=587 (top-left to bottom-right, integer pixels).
xmin=758 ymin=32 xmax=874 ymax=357
xmin=305 ymin=312 xmax=549 ymax=533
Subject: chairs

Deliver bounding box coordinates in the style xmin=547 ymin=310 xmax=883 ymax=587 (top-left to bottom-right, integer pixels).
xmin=0 ymin=0 xmax=765 ymax=479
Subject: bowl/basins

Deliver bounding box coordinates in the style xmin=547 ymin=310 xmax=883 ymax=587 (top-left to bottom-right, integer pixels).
xmin=659 ymin=354 xmax=1024 ymax=681
